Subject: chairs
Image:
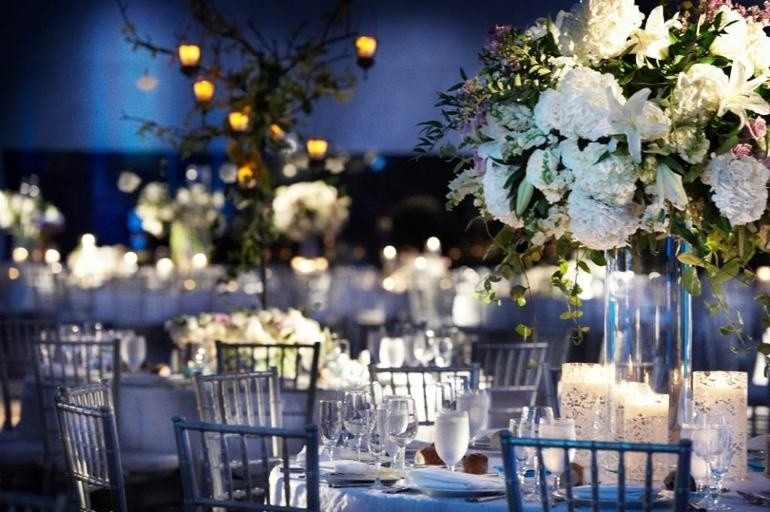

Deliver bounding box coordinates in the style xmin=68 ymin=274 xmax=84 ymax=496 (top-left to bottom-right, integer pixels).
xmin=0 ymin=223 xmax=770 ymax=512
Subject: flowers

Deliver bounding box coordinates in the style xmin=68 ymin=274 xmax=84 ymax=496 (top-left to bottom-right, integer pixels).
xmin=0 ymin=180 xmax=66 ymax=238
xmin=116 ymin=170 xmax=229 ymax=239
xmin=269 ymin=180 xmax=351 ymax=248
xmin=419 ymin=0 xmax=769 ymax=369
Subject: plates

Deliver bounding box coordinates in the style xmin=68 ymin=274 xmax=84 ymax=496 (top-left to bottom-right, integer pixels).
xmin=552 ymin=484 xmax=675 ymax=507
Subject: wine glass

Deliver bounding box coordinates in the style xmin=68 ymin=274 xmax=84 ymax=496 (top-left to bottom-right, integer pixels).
xmin=319 ymin=391 xmax=577 ymax=503
xmin=378 ymin=330 xmax=454 ymax=368
xmin=680 ymin=398 xmax=738 ymax=511
xmin=41 ymin=322 xmax=147 ymax=378
